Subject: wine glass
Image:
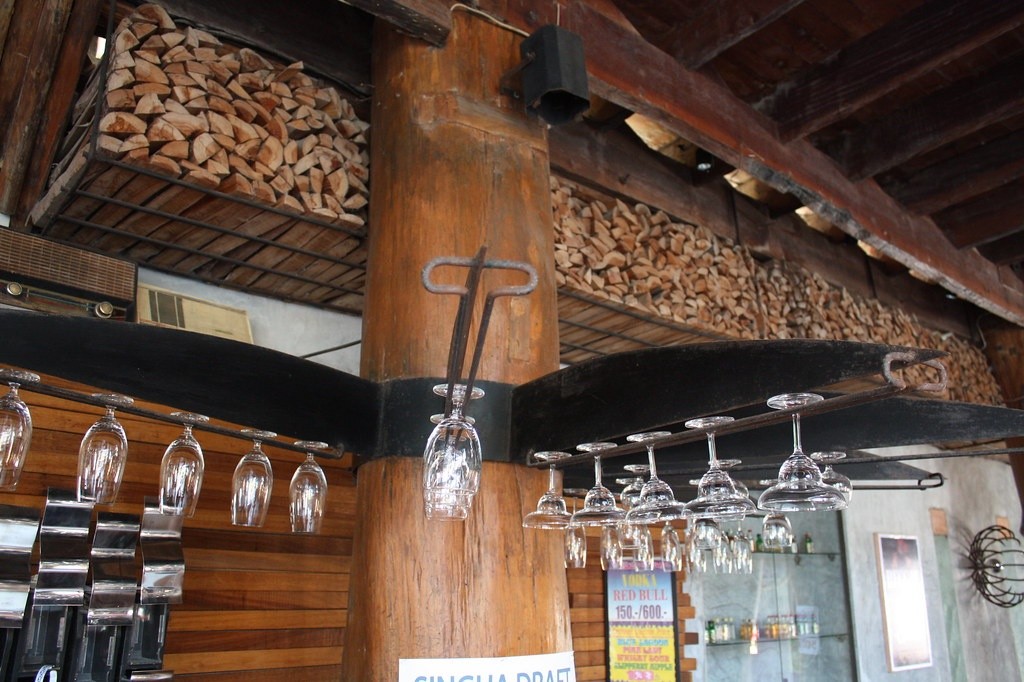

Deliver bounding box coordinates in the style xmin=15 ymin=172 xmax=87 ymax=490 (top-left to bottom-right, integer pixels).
xmin=422 ymin=383 xmax=486 ymax=521
xmin=0 ymin=368 xmax=41 ymax=491
xmin=159 ymin=412 xmax=210 ymax=518
xmin=77 ymin=393 xmax=134 ymax=507
xmin=230 ymin=429 xmax=277 ymax=528
xmin=289 ymin=441 xmax=328 ymax=533
xmin=523 ymin=392 xmax=852 ymax=574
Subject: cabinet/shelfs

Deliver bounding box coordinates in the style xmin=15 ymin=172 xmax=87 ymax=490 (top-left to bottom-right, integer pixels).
xmin=704 ymin=547 xmax=848 ymax=647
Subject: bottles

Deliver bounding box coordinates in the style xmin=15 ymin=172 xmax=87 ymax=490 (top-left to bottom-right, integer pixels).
xmin=705 ymin=613 xmax=821 ymax=643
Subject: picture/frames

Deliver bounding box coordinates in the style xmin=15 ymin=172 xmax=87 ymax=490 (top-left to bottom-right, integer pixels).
xmin=873 ymin=533 xmax=935 ymax=674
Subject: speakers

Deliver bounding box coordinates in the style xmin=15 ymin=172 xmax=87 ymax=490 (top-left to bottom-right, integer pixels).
xmin=0 ymin=223 xmax=139 ymax=322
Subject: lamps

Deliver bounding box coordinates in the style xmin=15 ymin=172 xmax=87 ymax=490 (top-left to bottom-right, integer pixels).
xmin=518 ymin=0 xmax=591 ymax=127
